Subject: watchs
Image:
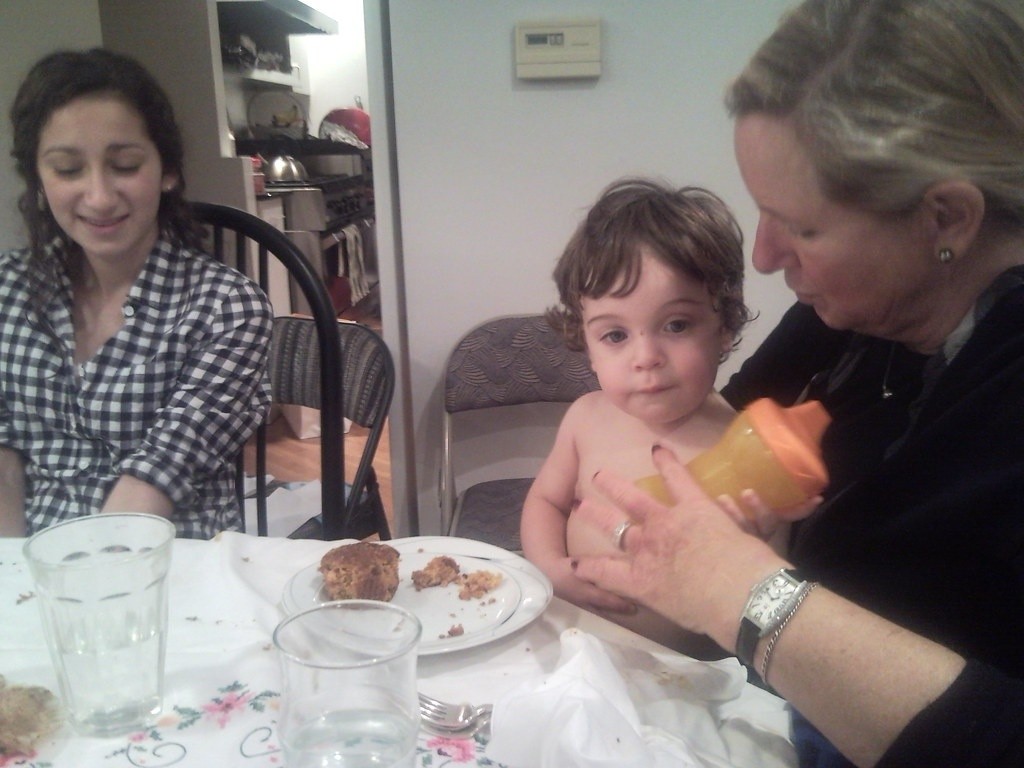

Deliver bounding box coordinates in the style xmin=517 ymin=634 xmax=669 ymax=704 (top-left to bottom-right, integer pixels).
xmin=734 ymin=565 xmax=808 ymax=687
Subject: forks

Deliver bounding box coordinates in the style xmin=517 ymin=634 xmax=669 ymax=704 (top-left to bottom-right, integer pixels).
xmin=394 ymin=692 xmax=494 ymax=740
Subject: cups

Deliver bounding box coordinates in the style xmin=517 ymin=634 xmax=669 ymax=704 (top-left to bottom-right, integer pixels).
xmin=23 ymin=512 xmax=176 ymax=740
xmin=273 ymin=600 xmax=422 ymax=768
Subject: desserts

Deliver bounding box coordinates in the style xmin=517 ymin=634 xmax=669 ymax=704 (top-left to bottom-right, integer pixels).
xmin=411 ymin=554 xmax=503 ymax=599
xmin=317 ymin=543 xmax=401 ymax=604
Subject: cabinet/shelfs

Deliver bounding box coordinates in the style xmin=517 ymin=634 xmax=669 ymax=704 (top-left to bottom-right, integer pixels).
xmin=259 ymin=34 xmax=311 ymax=98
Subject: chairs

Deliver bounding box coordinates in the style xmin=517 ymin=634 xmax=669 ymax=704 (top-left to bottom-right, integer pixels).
xmin=245 ymin=312 xmax=395 ymax=539
xmin=175 ymin=201 xmax=345 ymax=543
xmin=439 ymin=313 xmax=602 ymax=554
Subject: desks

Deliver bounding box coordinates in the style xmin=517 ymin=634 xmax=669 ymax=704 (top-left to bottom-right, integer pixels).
xmin=0 ymin=531 xmax=799 ymax=768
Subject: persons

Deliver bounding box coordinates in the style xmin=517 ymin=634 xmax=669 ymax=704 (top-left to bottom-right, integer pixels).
xmin=518 ymin=180 xmax=792 ymax=660
xmin=0 ymin=44 xmax=276 ymax=540
xmin=567 ymin=1 xmax=1022 ymax=766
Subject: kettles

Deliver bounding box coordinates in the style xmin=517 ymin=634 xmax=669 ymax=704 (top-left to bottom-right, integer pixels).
xmin=255 ymin=152 xmax=310 ymax=183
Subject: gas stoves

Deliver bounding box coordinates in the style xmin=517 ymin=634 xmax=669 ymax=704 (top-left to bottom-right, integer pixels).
xmin=265 ymin=174 xmax=373 ymax=230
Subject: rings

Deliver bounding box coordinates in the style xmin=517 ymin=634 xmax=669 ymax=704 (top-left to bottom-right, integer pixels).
xmin=612 ymin=520 xmax=637 ymax=548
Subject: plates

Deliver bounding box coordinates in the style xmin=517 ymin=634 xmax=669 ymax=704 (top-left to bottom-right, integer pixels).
xmin=281 ymin=535 xmax=554 ymax=655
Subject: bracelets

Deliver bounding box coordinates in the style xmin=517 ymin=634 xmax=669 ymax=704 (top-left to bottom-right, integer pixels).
xmin=760 ymin=581 xmax=821 ymax=689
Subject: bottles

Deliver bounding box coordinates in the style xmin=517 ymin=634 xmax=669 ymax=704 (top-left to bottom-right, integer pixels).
xmin=632 ymin=397 xmax=832 ymax=524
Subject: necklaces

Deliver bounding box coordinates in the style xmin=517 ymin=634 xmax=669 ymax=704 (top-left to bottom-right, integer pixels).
xmin=882 ymin=341 xmax=900 ymax=398
